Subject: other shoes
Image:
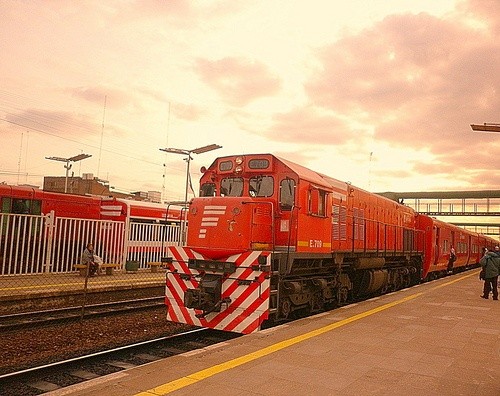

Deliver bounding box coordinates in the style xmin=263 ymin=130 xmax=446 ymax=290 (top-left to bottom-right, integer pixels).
xmin=480 ymin=295 xmax=488 ymax=299
xmin=89 ymin=272 xmax=94 ymax=277
xmin=493 ymin=296 xmax=498 ymax=300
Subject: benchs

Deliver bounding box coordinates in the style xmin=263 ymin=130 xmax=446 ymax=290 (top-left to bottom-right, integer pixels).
xmin=74 ymin=263 xmax=120 ymax=276
xmin=149 ymin=262 xmax=167 ymax=273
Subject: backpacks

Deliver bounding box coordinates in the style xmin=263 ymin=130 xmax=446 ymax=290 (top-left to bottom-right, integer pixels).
xmin=450 ymin=255 xmax=456 ymax=263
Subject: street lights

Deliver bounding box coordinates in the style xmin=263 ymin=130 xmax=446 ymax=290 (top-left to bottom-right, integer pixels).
xmin=45 ymin=153 xmax=92 ymax=194
xmin=159 ymin=144 xmax=223 ymax=246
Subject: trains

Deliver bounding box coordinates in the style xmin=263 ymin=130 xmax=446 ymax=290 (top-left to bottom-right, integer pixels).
xmin=0 ymin=184 xmax=189 ymax=275
xmin=165 ymin=153 xmax=500 ymax=334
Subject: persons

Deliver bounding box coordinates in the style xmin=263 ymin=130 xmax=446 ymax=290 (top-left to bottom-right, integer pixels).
xmin=447 ymin=244 xmax=455 ymax=274
xmin=82 ymin=243 xmax=99 ymax=277
xmin=479 ymin=246 xmax=500 ymax=301
